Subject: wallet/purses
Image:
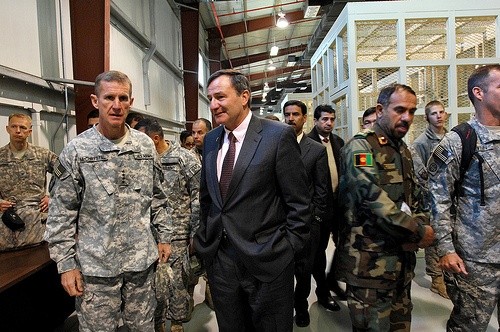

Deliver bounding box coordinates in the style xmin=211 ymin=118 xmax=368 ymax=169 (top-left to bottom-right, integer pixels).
xmin=1 ymin=206 xmax=26 ymax=231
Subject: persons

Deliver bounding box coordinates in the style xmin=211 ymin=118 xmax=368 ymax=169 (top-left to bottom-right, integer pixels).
xmin=426 ymin=64 xmax=500 ymax=332
xmin=187 ymin=118 xmax=215 ymax=311
xmin=0 ymin=113 xmax=59 ymax=251
xmin=338 ymin=83 xmax=434 ymax=332
xmin=305 ymin=104 xmax=377 ymax=311
xmin=411 ymin=100 xmax=449 ymax=300
xmin=132 ymin=118 xmax=202 ymax=332
xmin=193 ymin=70 xmax=313 ymax=332
xmin=283 ymin=100 xmax=329 ymax=327
xmin=87 ymin=111 xmax=280 ymax=151
xmin=43 ymin=70 xmax=174 ymax=332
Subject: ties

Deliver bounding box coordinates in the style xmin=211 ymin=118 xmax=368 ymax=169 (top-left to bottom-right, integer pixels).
xmin=220 ymin=132 xmax=237 ymax=205
xmin=323 ymin=137 xmax=329 ymax=143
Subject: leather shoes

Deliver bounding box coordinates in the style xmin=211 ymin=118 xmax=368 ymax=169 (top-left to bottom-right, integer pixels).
xmin=327 ymin=279 xmax=345 ymax=297
xmin=296 ymin=310 xmax=310 ymax=327
xmin=318 ymin=296 xmax=341 ymax=311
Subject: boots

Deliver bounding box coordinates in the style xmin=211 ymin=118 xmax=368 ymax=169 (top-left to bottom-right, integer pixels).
xmin=204 ymin=288 xmax=215 ymax=310
xmin=430 ymin=275 xmax=449 ymax=298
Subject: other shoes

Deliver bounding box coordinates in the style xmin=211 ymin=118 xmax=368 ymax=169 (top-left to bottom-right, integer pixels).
xmin=170 ymin=322 xmax=184 ymax=332
xmin=155 ymin=322 xmax=166 ymax=332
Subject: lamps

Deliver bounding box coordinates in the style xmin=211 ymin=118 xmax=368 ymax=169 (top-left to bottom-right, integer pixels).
xmin=276 ymin=11 xmax=288 ymax=29
